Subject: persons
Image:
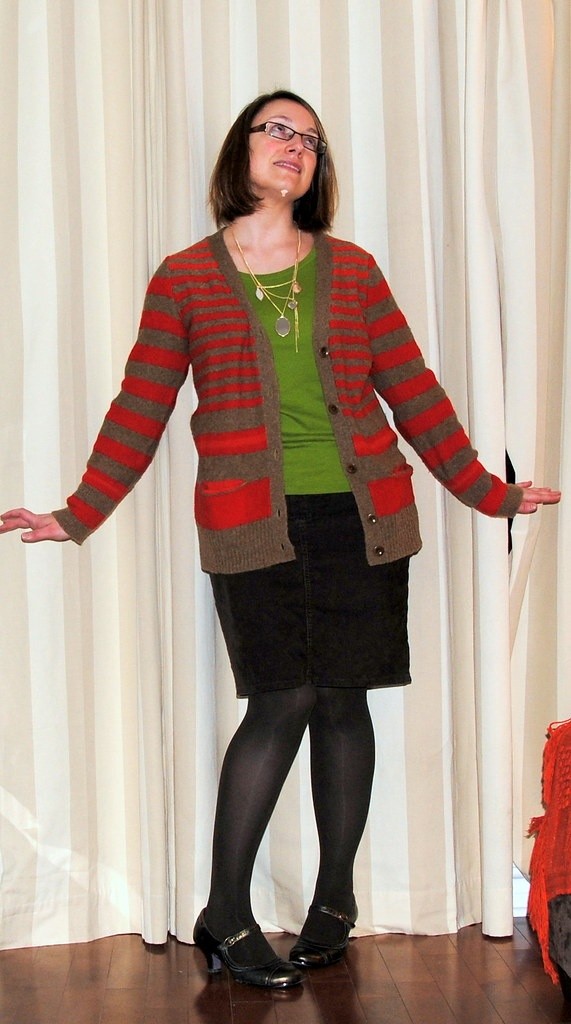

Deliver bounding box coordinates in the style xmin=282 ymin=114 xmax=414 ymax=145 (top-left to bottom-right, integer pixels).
xmin=0 ymin=91 xmax=561 ymax=987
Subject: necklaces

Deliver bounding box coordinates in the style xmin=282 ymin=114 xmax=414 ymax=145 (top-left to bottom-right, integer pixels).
xmin=232 ymin=224 xmax=303 ymax=355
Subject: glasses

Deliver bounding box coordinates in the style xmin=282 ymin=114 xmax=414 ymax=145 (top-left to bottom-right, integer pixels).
xmin=247 ymin=119 xmax=327 ymax=155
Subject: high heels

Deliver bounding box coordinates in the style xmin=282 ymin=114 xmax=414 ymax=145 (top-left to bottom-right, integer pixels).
xmin=193 ymin=908 xmax=303 ymax=989
xmin=289 ymin=904 xmax=356 ymax=968
